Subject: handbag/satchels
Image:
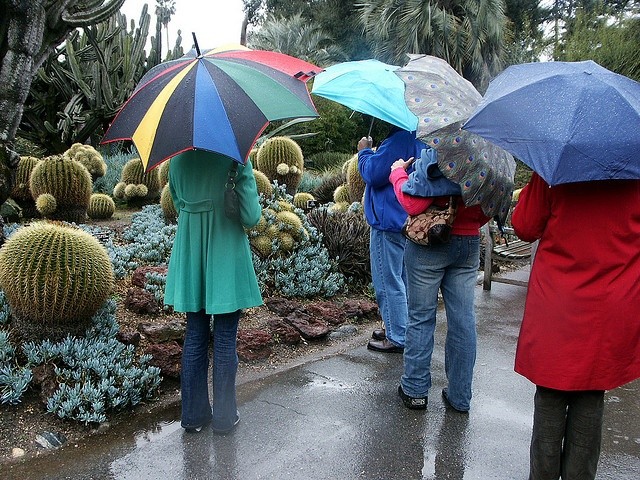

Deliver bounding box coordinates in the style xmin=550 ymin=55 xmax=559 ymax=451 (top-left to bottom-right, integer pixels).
xmin=402 ymin=195 xmax=457 ymax=248
xmin=223 ymin=160 xmax=242 ymax=222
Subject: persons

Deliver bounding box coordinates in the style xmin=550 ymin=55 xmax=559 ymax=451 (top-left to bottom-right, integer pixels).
xmin=163 ymin=151 xmax=264 ymax=433
xmin=511 ymin=172 xmax=640 ymax=480
xmin=357 ymin=131 xmax=430 ymax=353
xmin=389 ymin=148 xmax=492 ymax=413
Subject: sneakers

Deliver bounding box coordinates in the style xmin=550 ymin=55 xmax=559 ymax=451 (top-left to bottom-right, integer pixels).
xmin=398 ymin=384 xmax=429 ymax=410
xmin=233 ymin=409 xmax=240 ymax=426
xmin=443 ymin=387 xmax=468 ymax=414
xmin=185 ymin=409 xmax=214 ymax=432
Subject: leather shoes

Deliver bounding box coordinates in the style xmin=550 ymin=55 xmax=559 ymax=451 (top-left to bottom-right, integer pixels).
xmin=372 ymin=330 xmax=386 ymax=339
xmin=368 ymin=340 xmax=404 ymax=354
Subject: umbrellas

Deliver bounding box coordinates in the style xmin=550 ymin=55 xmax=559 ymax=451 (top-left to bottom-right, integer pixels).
xmin=309 ymin=59 xmax=418 ymax=138
xmin=393 ymin=53 xmax=516 ymax=234
xmin=459 ymin=60 xmax=640 ymax=189
xmin=99 ymin=32 xmax=326 ymax=174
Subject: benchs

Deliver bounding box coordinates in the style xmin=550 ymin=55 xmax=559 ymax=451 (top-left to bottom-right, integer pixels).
xmin=483 ymin=201 xmax=540 ymax=292
